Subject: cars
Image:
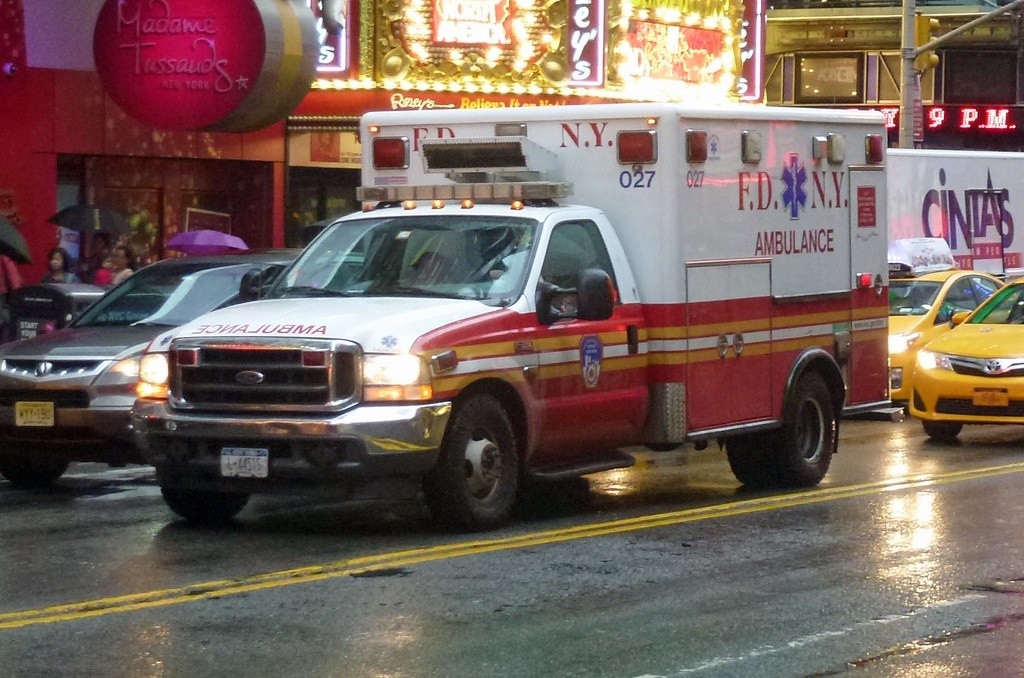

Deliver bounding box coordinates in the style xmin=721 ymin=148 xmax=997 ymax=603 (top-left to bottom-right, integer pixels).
xmin=879 ymin=237 xmax=1011 ymax=406
xmin=917 ymin=278 xmax=1023 ymax=440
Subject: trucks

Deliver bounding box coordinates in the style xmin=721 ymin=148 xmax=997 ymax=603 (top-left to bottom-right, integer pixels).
xmin=886 ymin=150 xmax=1023 ymax=284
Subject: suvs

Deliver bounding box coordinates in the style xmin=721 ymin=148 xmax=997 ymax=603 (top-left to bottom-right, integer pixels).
xmin=2 ymin=251 xmax=365 ymax=487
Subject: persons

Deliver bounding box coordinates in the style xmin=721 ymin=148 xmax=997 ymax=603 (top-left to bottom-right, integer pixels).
xmin=479 ymin=225 xmax=543 ymax=303
xmin=0 ymin=256 xmax=21 ymax=323
xmin=76 ymin=231 xmax=138 ymax=288
xmin=42 ymin=248 xmax=80 ymax=284
xmin=404 ymin=251 xmax=443 ymax=286
xmin=922 ymin=286 xmax=954 ymax=320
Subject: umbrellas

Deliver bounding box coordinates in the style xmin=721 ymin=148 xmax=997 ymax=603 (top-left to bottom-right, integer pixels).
xmin=165 ymin=229 xmax=248 ymax=256
xmin=299 ymin=218 xmax=341 ymax=244
xmin=0 ymin=219 xmax=31 ymax=264
xmin=50 ymin=204 xmax=132 ymax=256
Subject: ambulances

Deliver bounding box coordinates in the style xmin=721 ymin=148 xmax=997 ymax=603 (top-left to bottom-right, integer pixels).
xmin=132 ymin=101 xmax=894 ymax=535
